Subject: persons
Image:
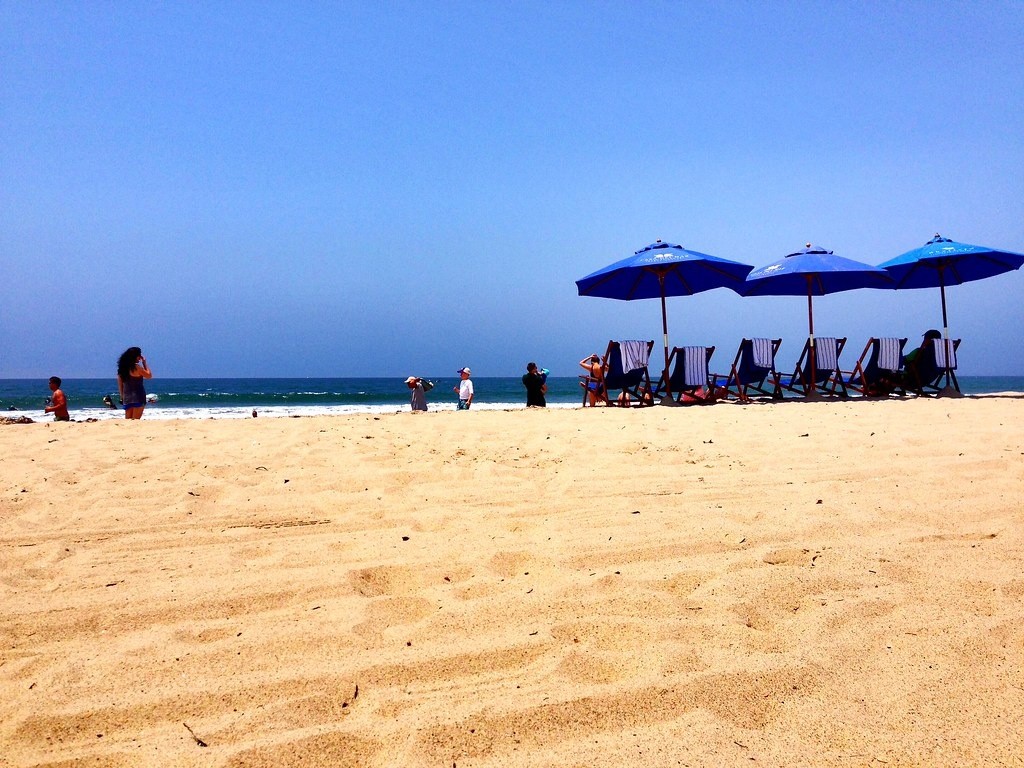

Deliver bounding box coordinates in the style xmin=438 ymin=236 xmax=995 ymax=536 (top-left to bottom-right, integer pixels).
xmin=889 ymin=330 xmax=941 ymax=394
xmin=522 ymin=363 xmax=550 ymax=407
xmin=404 ymin=376 xmax=428 ymax=411
xmin=45 ymin=347 xmax=151 ymax=421
xmin=453 ymin=367 xmax=473 ymax=411
xmin=579 ymin=353 xmax=609 ymax=402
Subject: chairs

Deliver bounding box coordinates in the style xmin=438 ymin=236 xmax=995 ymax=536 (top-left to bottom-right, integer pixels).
xmin=579 ymin=336 xmax=962 ymax=407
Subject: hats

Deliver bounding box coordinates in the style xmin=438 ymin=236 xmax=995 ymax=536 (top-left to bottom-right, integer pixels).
xmin=921 ymin=329 xmax=941 ymax=339
xmin=541 ymin=368 xmax=549 ymax=376
xmin=456 ymin=367 xmax=470 ymax=374
xmin=404 ymin=376 xmax=415 ymax=384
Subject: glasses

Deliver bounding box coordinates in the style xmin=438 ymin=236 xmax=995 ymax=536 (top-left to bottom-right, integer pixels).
xmin=49 ymin=382 xmax=54 ymax=384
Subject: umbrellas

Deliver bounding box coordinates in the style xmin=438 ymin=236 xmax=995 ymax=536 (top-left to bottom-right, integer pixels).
xmin=575 ymin=239 xmax=755 ymax=398
xmin=740 ymin=244 xmax=891 ymax=390
xmin=877 ymin=232 xmax=1024 ymax=338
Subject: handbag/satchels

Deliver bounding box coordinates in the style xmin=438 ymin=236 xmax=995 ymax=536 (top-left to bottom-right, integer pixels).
xmin=419 ymin=378 xmax=433 ymax=392
